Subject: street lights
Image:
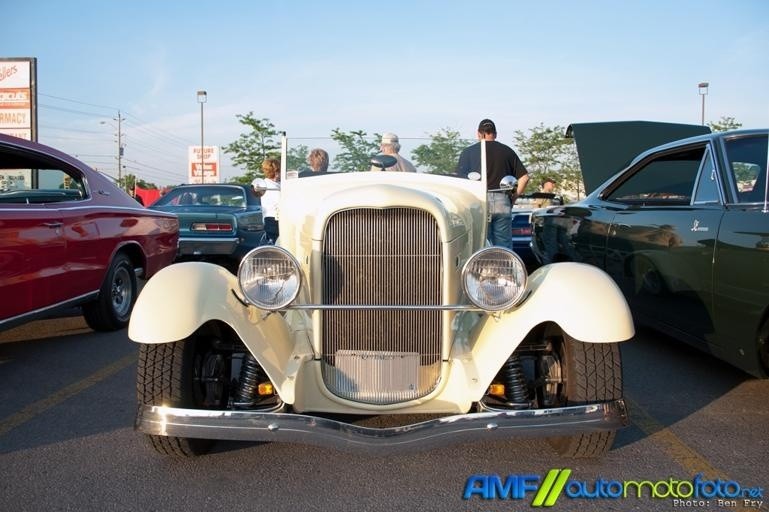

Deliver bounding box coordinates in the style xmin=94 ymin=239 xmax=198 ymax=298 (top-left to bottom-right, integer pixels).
xmin=100 ymin=121 xmax=121 ymax=188
xmin=197 ymin=91 xmax=207 ymax=183
xmin=699 ymin=83 xmax=708 ymax=126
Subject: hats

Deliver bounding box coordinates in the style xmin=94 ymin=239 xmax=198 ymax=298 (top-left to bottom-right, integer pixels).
xmin=382 ymin=133 xmax=399 ymax=145
xmin=542 ymin=178 xmax=557 ymax=187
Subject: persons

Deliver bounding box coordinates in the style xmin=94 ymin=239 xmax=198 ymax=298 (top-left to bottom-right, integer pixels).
xmin=371 ymin=132 xmax=416 ymax=172
xmin=533 ymin=179 xmax=556 ymax=208
xmin=309 ymin=149 xmax=329 ymax=172
xmin=458 ymin=119 xmax=530 ymax=251
xmin=251 ymin=160 xmax=281 ymax=220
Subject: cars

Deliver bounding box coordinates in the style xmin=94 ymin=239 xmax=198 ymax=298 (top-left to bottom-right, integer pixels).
xmin=127 ymin=133 xmax=635 ymax=460
xmin=147 ymin=183 xmax=269 ymax=275
xmin=0 ymin=133 xmax=179 ymax=331
xmin=532 ymin=123 xmax=768 ymax=378
xmin=511 ymin=192 xmax=564 ymax=264
